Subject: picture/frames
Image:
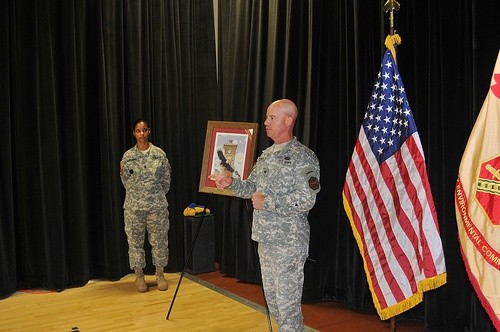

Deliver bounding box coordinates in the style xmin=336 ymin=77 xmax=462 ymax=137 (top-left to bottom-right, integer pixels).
xmin=199 ymin=121 xmax=258 ymax=196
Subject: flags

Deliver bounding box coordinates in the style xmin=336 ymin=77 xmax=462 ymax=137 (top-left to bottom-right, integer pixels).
xmin=342 ymin=35 xmax=447 ymax=321
xmin=455 ymin=51 xmax=500 ymax=332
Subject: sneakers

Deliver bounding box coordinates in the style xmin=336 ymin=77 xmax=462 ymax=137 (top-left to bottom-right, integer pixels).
xmin=156 ymin=275 xmax=168 ymax=291
xmin=135 ymin=275 xmax=148 ymax=292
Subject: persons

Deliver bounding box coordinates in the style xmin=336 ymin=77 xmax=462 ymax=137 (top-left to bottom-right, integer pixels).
xmin=120 ymin=121 xmax=170 ymax=292
xmin=208 ymin=98 xmax=320 ymax=332
